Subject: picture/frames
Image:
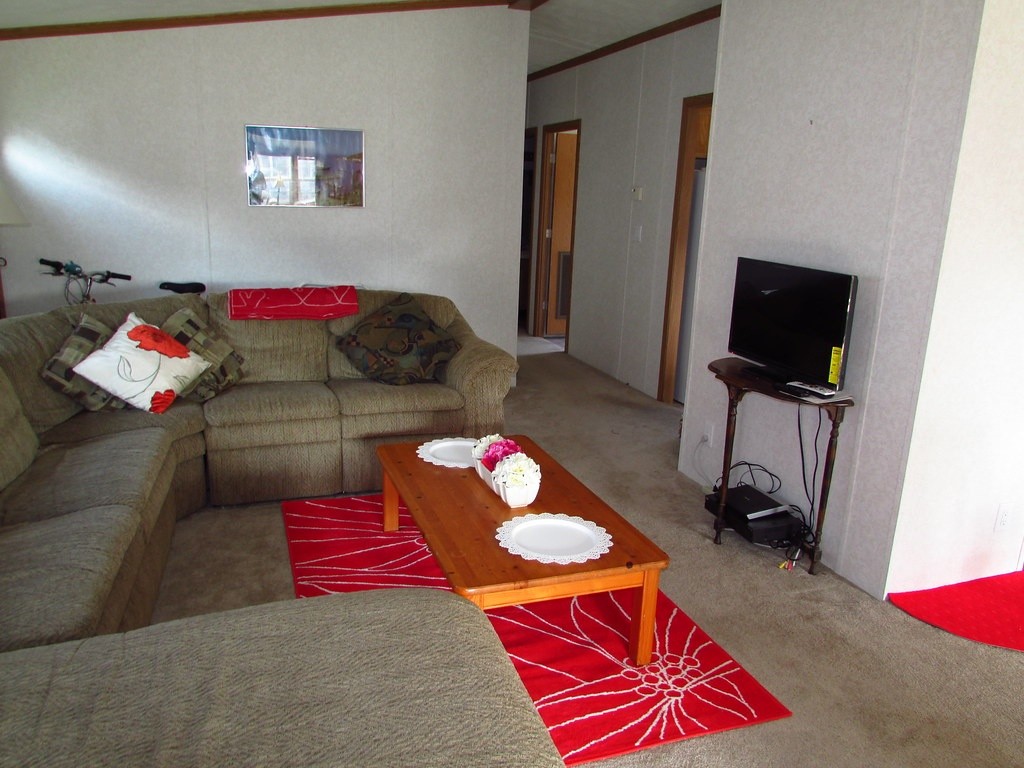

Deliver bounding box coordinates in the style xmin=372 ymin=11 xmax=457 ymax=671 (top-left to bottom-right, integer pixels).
xmin=244 ymin=124 xmax=365 ymax=207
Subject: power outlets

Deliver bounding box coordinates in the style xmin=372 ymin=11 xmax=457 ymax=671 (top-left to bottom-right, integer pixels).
xmin=702 ymin=421 xmax=713 ymax=448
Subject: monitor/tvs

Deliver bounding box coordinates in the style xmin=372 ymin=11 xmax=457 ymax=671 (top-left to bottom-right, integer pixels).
xmin=728 ymin=257 xmax=858 ymax=391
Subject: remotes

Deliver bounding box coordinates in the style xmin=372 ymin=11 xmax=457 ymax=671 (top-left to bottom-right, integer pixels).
xmin=775 ymin=381 xmax=836 ymax=399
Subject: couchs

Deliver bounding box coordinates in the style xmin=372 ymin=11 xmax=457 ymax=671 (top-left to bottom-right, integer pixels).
xmin=0 ymin=290 xmax=564 ymax=768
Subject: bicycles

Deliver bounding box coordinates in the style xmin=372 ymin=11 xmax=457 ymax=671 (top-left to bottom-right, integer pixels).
xmin=38 ymin=258 xmax=206 ymax=304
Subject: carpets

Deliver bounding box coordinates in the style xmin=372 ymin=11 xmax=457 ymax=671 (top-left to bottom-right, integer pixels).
xmin=282 ymin=495 xmax=794 ymax=767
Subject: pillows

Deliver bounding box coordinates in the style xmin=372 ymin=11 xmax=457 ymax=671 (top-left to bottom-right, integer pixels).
xmin=40 ymin=311 xmax=134 ymax=413
xmin=160 ymin=306 xmax=251 ymax=403
xmin=74 ymin=310 xmax=211 ymax=414
xmin=335 ymin=291 xmax=463 ymax=385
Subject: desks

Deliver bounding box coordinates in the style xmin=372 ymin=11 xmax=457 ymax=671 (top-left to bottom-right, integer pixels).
xmin=707 ymin=357 xmax=853 ymax=575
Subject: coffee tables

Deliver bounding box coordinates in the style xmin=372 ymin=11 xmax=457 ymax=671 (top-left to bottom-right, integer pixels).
xmin=377 ymin=435 xmax=669 ymax=667
